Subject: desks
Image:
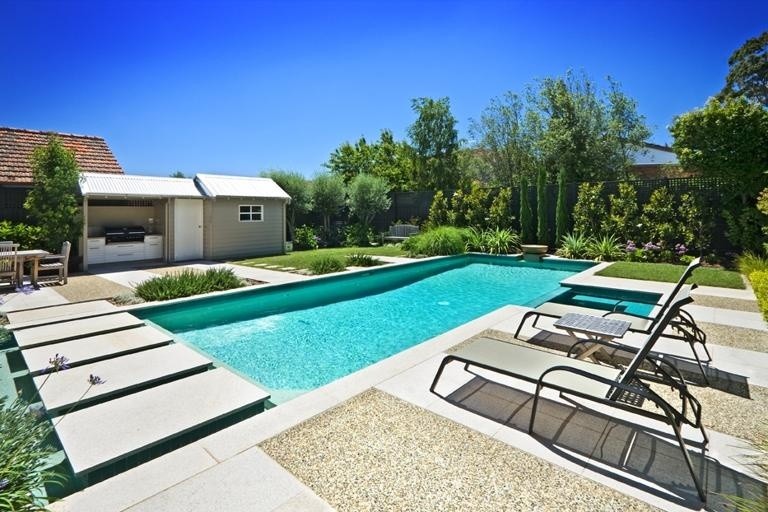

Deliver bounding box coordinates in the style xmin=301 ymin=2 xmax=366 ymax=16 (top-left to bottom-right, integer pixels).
xmin=0 ymin=249 xmax=49 ymax=289
xmin=555 ymin=313 xmax=631 ymax=365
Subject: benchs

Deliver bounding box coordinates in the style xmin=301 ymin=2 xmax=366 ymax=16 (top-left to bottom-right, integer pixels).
xmin=381 ymin=224 xmax=419 ymax=245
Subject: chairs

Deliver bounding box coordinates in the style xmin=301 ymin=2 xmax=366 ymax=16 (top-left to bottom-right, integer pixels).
xmin=37 ymin=241 xmax=70 ymax=284
xmin=0 ymin=244 xmax=21 ymax=290
xmin=428 ymin=284 xmax=709 ymax=502
xmin=515 ymin=255 xmax=712 ymax=382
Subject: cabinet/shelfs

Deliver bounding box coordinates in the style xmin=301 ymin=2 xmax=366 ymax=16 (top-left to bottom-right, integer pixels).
xmin=87 ymin=233 xmax=165 ymax=265
